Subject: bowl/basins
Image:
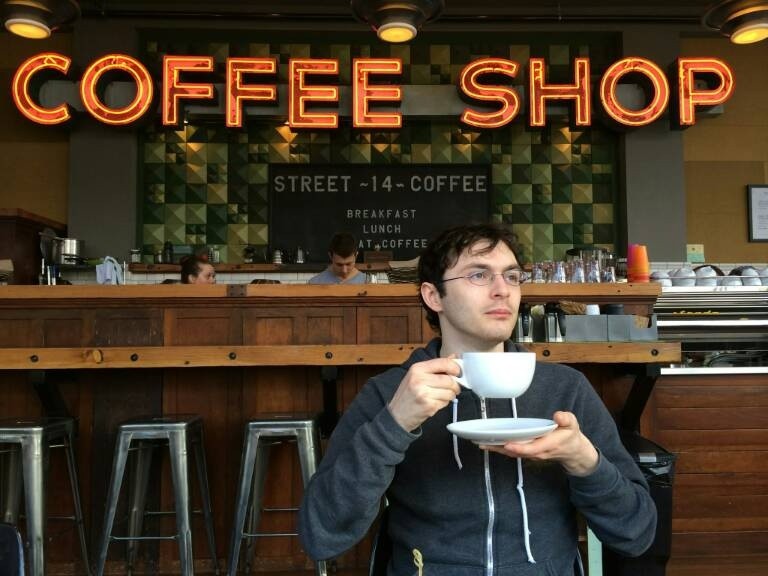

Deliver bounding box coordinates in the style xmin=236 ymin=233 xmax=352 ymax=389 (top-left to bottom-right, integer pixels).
xmin=650 ymin=267 xmax=768 ymax=287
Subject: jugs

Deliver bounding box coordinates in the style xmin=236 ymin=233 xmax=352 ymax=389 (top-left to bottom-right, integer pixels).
xmin=512 ymin=303 xmax=535 ymax=342
xmin=543 ymin=302 xmax=566 ymax=342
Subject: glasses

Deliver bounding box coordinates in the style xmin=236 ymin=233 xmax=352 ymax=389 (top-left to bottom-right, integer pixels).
xmin=434 ymin=270 xmax=529 ymax=286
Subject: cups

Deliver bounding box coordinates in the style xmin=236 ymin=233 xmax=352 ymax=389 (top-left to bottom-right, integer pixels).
xmin=626 ymin=243 xmax=650 ymax=284
xmin=531 ymin=250 xmax=617 ymax=283
xmin=446 ymin=352 xmax=536 ymax=399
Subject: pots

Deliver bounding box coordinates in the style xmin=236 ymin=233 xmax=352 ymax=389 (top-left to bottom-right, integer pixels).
xmin=38 ymin=232 xmax=85 ymax=265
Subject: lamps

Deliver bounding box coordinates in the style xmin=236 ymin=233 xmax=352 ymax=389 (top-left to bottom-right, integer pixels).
xmin=1 ymin=0 xmax=81 ymax=40
xmin=699 ymin=0 xmax=768 ymax=44
xmin=350 ymin=0 xmax=445 ymax=42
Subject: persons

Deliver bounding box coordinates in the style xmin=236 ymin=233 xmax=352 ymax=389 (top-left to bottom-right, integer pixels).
xmin=297 ymin=227 xmax=659 ymax=576
xmin=308 ymin=232 xmax=370 ymax=284
xmin=176 ymin=254 xmax=217 ymax=285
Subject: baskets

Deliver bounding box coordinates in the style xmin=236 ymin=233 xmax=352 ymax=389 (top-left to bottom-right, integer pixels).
xmin=387 ymin=267 xmax=420 ymax=283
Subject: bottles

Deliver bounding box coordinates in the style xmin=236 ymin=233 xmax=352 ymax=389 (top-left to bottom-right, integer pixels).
xmin=131 ymin=249 xmax=141 ymax=264
xmin=164 ymin=241 xmax=173 ymax=264
xmin=157 ymin=250 xmax=163 ymax=264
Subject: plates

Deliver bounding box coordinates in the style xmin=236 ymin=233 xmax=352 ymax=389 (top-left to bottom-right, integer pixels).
xmin=446 ymin=418 xmax=559 ymax=446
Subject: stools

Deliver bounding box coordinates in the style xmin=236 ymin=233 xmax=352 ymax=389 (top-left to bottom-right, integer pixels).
xmin=98 ymin=413 xmax=222 ymax=576
xmin=0 ymin=416 xmax=93 ymax=576
xmin=231 ymin=411 xmax=336 ymax=576
